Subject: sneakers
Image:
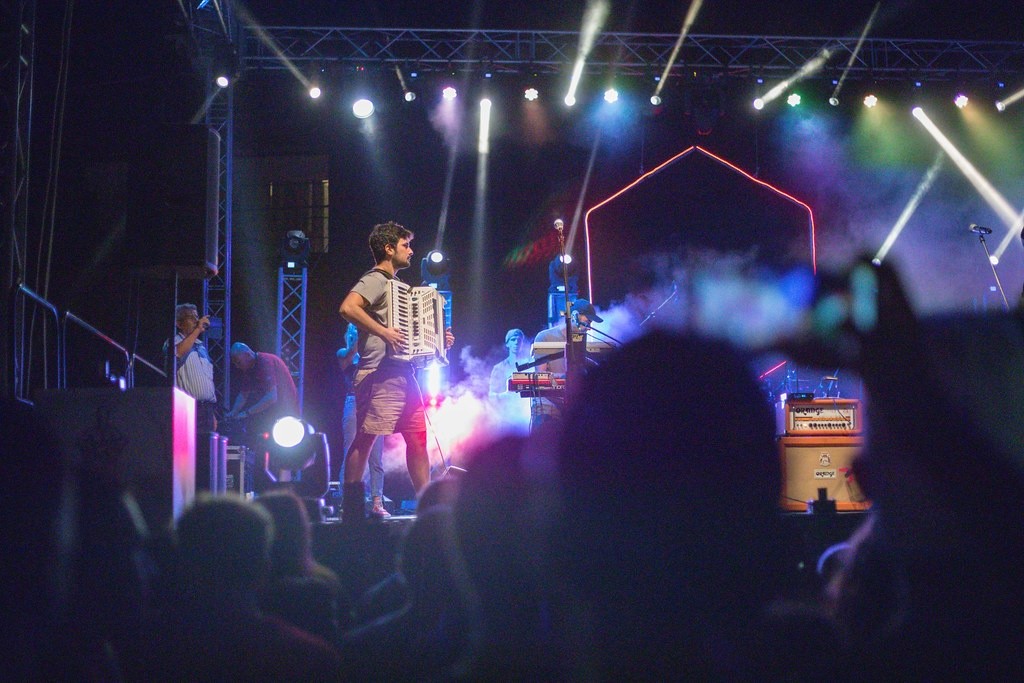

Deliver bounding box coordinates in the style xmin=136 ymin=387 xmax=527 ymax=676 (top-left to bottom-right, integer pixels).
xmin=371 ymin=505 xmax=391 ymax=518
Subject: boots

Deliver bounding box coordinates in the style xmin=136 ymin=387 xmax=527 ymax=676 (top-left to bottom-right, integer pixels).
xmin=342 ymin=481 xmax=384 ymax=526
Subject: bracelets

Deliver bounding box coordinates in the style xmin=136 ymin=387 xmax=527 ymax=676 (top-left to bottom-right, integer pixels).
xmin=244 ymin=408 xmax=249 ymax=415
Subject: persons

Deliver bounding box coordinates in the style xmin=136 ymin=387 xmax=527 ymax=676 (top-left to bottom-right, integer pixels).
xmin=161 ymin=304 xmax=217 ymax=499
xmin=225 ymin=342 xmax=301 ymax=498
xmin=488 ymin=329 xmax=530 ymax=421
xmin=339 ymin=220 xmax=456 ymax=514
xmin=1 ymin=251 xmax=1024 ymax=683
xmin=337 ymin=322 xmax=391 ymax=519
xmin=531 ymin=299 xmax=603 ymax=432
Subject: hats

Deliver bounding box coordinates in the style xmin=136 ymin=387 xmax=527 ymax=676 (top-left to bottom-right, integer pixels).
xmin=570 ymin=299 xmax=603 ymax=323
xmin=505 ymin=329 xmax=524 ymax=343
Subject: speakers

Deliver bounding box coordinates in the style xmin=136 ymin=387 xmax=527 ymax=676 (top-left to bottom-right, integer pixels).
xmin=777 ymin=436 xmax=873 ymax=512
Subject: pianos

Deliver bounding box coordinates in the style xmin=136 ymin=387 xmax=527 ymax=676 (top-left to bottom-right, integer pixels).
xmin=510 ymin=339 xmax=620 ymax=426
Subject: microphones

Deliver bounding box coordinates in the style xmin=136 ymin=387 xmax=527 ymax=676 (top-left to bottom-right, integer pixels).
xmin=673 ymin=281 xmax=678 ymax=302
xmin=553 ymin=219 xmax=564 ymax=231
xmin=968 ymin=224 xmax=992 ymax=234
xmin=572 ymin=310 xmax=581 ymax=331
